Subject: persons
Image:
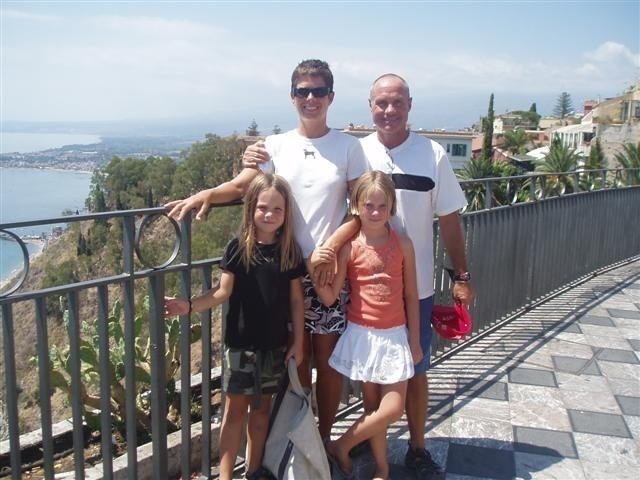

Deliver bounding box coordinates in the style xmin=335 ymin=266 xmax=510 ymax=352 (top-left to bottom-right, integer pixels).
xmin=162 ymin=54 xmax=380 ymax=453
xmin=305 ymin=170 xmax=426 ymax=480
xmin=242 ymin=73 xmax=477 ymax=480
xmin=166 ymin=173 xmax=307 ymax=480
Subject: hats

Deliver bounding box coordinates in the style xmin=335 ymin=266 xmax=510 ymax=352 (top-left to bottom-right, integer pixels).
xmin=430 ymin=303 xmax=473 ymax=339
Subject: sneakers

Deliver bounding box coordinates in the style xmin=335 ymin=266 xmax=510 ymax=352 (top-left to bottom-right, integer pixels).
xmin=405 ymin=446 xmax=444 ymax=480
xmin=243 ymin=467 xmax=277 ymax=480
xmin=349 ymin=438 xmax=371 ymax=458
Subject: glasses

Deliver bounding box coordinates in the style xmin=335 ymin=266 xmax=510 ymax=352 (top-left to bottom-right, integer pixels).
xmin=291 ymin=87 xmax=332 ymax=98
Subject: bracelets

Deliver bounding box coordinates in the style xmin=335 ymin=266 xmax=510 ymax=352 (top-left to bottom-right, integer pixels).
xmin=187 ymin=299 xmax=192 ymax=315
xmin=452 ymin=271 xmax=473 ymax=284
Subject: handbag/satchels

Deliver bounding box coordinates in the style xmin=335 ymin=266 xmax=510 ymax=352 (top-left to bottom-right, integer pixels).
xmin=263 ymin=358 xmax=332 ymax=479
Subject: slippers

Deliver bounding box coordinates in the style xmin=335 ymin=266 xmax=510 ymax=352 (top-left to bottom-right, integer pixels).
xmin=324 ymin=440 xmax=392 ymax=480
